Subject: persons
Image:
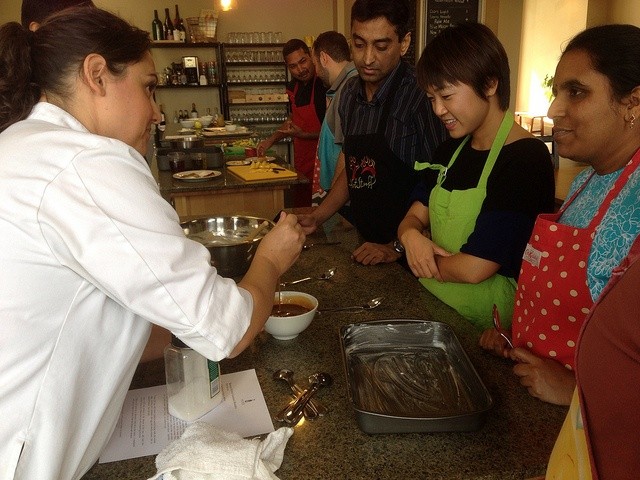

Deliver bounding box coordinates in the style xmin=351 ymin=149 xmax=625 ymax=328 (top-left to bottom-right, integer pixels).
xmin=1 ymin=7 xmax=307 ymax=480
xmin=531 ymin=225 xmax=640 ymax=479
xmin=396 ymin=21 xmax=556 ymax=332
xmin=257 ymin=38 xmax=331 ymax=198
xmin=298 ymin=1 xmax=466 ymax=266
xmin=478 ymin=24 xmax=639 ymax=406
xmin=312 ymin=30 xmax=358 ymax=210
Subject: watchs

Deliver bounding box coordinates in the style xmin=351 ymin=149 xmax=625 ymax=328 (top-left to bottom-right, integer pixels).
xmin=392 ymin=240 xmax=403 ymax=258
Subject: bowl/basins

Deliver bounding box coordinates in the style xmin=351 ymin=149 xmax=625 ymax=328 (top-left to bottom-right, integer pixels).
xmin=181 ymin=215 xmax=277 ymax=279
xmin=201 ymin=116 xmax=213 ymax=121
xmin=202 ymin=121 xmax=211 ymax=127
xmin=180 ymin=121 xmax=195 ymax=128
xmin=171 ymin=140 xmax=200 ymax=150
xmin=264 ymin=291 xmax=319 ymax=340
xmin=225 ymin=125 xmax=236 ymax=132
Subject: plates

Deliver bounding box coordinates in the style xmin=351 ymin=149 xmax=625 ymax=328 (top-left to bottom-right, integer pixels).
xmin=173 ymin=169 xmax=222 ymax=183
xmin=246 ymin=157 xmax=276 ymax=162
xmin=202 ymin=127 xmax=254 ymax=137
xmin=204 ymin=128 xmax=224 ymax=132
xmin=227 ymin=161 xmax=251 ymax=166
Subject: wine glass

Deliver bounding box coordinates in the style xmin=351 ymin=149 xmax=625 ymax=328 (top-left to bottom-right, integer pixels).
xmin=226 ymin=48 xmax=258 ymax=62
xmin=226 ymin=69 xmax=287 ymax=82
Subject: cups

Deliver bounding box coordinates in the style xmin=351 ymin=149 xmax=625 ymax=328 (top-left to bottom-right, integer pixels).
xmin=228 ymin=32 xmax=284 ymax=44
xmin=229 ymin=108 xmax=288 ymax=125
xmin=259 ymin=49 xmax=285 ymax=63
xmin=190 ymin=152 xmax=204 ymax=171
xmin=167 ymin=152 xmax=185 ymax=173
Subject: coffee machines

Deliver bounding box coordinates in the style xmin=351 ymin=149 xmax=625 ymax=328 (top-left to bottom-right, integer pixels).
xmin=182 ymin=56 xmax=200 ymax=86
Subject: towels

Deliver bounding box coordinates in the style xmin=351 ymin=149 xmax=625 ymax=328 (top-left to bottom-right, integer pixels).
xmin=146 ymin=418 xmax=294 ymax=480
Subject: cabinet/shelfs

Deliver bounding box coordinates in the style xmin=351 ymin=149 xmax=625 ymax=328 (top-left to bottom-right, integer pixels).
xmin=144 ymin=40 xmax=226 ymax=147
xmin=222 ymin=41 xmax=293 ymax=165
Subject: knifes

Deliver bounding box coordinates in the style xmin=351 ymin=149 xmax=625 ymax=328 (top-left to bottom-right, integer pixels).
xmin=253 ymin=168 xmax=285 ymax=170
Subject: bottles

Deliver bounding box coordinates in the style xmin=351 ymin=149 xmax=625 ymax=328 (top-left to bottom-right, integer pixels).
xmin=180 ymin=19 xmax=185 ymax=43
xmin=164 ymin=8 xmax=174 ymax=40
xmin=183 ymin=110 xmax=189 ymax=119
xmin=206 ymin=108 xmax=211 ymax=117
xmin=179 ymin=109 xmax=184 ymax=120
xmin=152 ymin=10 xmax=162 ymax=41
xmin=173 ymin=111 xmax=178 ymax=123
xmin=158 ymin=104 xmax=165 ymax=130
xmin=163 ymin=333 xmax=225 ymax=423
xmin=190 ymin=103 xmax=198 ymax=118
xmin=175 ymin=4 xmax=181 ymax=42
xmin=214 ymin=107 xmax=219 ymax=119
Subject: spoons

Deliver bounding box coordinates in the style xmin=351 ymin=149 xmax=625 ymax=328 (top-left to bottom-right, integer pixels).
xmin=274 ymin=369 xmax=331 ymax=423
xmin=316 ymin=296 xmax=387 ymax=313
xmin=492 ymin=303 xmax=517 ymax=347
xmin=298 ymin=240 xmax=341 ymax=249
xmin=279 ymin=267 xmax=337 ymax=288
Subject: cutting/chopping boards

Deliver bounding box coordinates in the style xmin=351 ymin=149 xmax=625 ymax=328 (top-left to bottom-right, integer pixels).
xmin=227 ymin=162 xmax=298 ymax=182
